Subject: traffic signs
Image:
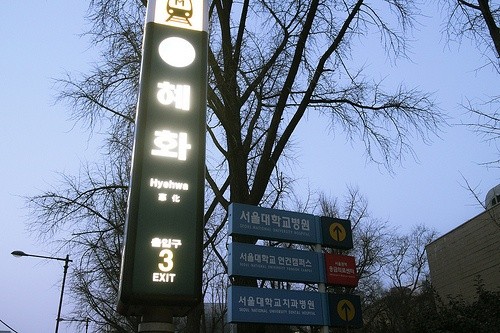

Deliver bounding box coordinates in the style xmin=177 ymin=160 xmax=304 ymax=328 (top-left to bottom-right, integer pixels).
xmin=230 ymin=242 xmax=319 ymax=276
xmin=230 ymin=286 xmax=323 ymax=328
xmin=230 ymin=206 xmax=317 ymax=244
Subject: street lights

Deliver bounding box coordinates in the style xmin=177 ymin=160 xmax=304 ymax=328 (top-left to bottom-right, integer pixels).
xmin=12 ymin=249 xmax=73 ymax=332
